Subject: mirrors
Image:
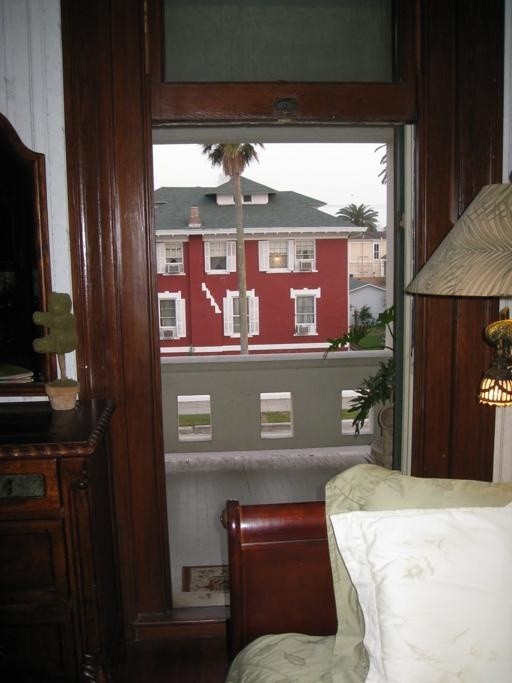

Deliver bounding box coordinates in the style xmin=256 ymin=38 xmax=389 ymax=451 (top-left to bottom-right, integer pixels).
xmin=0 ymin=109 xmax=60 ymax=400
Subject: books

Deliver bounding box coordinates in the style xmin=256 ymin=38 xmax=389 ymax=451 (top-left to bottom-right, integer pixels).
xmin=1 ymin=381 xmax=79 ymax=403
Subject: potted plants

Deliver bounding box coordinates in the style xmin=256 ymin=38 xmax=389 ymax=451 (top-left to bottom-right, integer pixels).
xmin=30 ymin=289 xmax=81 ymax=415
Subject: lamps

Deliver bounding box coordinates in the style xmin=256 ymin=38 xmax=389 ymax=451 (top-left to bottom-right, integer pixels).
xmin=400 ymin=182 xmax=512 ymax=408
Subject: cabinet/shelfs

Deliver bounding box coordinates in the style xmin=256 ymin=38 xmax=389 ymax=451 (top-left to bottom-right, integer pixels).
xmin=0 ymin=442 xmax=121 ymax=683
